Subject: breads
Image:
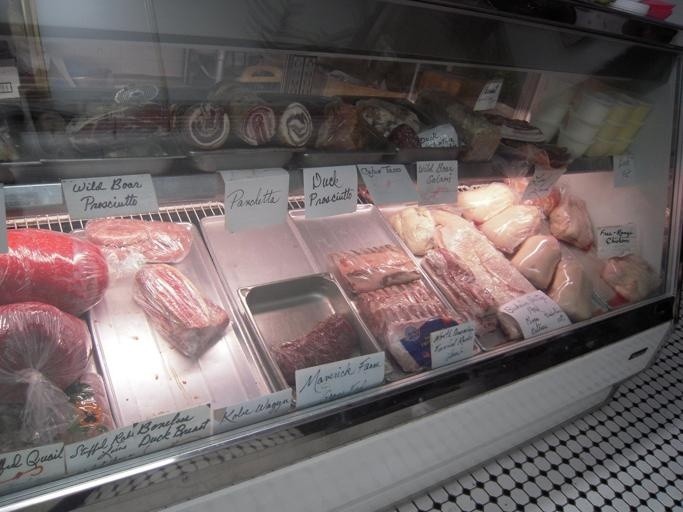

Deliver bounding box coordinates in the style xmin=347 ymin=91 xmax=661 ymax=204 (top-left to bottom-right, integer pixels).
xmin=0 ymin=91 xmax=553 ymax=168
